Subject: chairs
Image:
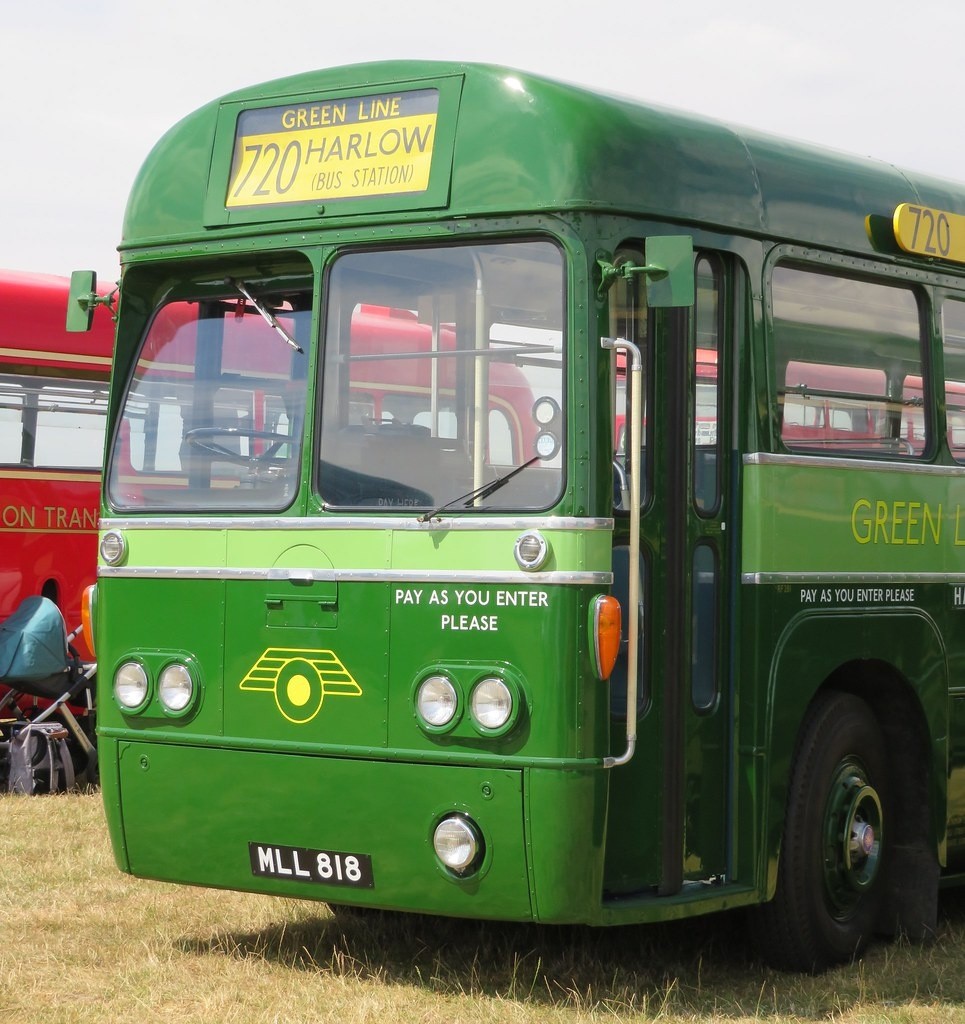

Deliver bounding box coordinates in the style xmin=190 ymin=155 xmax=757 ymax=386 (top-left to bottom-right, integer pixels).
xmin=335 ymin=426 xmax=431 ymax=481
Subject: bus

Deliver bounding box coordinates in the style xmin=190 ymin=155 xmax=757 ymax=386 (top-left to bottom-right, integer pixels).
xmin=65 ymin=58 xmax=965 ymax=975
xmin=0 ymin=268 xmax=965 ymax=800
